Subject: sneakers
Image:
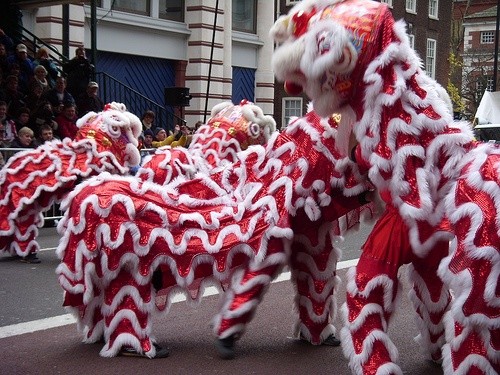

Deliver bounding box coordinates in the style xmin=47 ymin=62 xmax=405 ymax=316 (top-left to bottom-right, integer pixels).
xmin=25 ymin=254 xmax=41 ymax=263
xmin=12 ymin=255 xmax=22 ymax=260
xmin=323 ymin=333 xmax=340 ymax=346
xmin=120 ymin=342 xmax=169 ymax=357
xmin=216 ymin=335 xmax=239 ymax=359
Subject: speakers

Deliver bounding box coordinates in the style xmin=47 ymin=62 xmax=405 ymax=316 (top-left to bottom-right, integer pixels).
xmin=164 ymin=86 xmax=189 ymax=105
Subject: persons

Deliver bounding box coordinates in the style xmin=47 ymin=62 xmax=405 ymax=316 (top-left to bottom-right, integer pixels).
xmin=1 ymin=27 xmax=206 ymax=227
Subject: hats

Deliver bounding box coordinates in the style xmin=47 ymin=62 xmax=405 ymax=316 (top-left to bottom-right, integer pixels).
xmin=144 ymin=129 xmax=154 ymax=136
xmin=16 ymin=43 xmax=27 ymax=53
xmin=88 ymin=82 xmax=98 ymax=88
xmin=154 ymin=127 xmax=162 ymax=136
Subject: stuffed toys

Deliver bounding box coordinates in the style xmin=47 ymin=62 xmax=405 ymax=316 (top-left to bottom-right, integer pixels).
xmin=268 ymin=0 xmax=500 ymax=375
xmin=0 ymin=100 xmax=277 ymax=266
xmin=57 ymin=100 xmax=377 ymax=361
